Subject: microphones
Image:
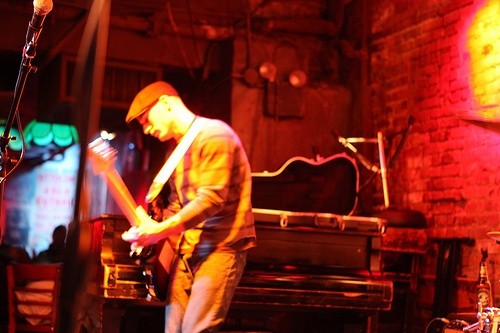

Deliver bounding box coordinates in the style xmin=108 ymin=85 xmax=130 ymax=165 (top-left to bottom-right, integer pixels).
xmin=14 ymin=0 xmax=53 ymax=99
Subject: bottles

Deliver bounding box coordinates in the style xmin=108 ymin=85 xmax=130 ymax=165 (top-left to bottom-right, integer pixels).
xmin=477 ymin=262 xmax=492 ymax=313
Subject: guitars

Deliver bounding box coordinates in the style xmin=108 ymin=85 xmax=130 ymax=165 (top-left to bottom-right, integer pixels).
xmin=81 ymin=129 xmax=174 ymax=303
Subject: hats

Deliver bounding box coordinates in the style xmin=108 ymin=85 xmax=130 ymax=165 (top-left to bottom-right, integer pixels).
xmin=125 ymin=80 xmax=179 ymax=125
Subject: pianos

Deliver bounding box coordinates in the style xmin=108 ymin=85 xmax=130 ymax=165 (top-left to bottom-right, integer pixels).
xmin=69 ymin=206 xmax=396 ymax=333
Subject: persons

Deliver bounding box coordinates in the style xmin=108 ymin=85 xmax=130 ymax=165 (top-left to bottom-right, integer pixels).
xmin=121 ymin=80 xmax=260 ymax=333
xmin=30 ymin=225 xmax=66 ymax=264
